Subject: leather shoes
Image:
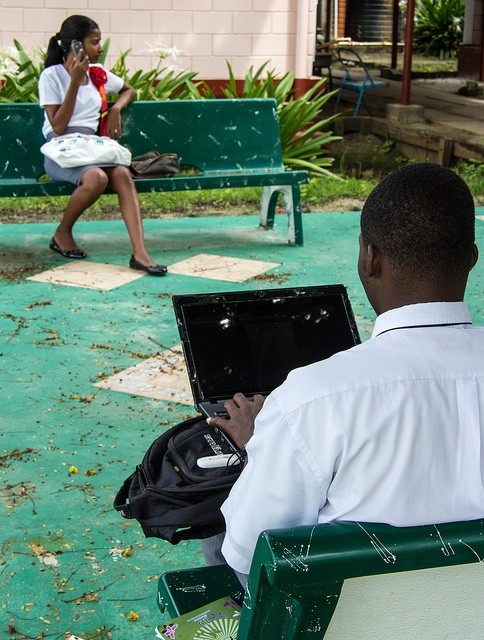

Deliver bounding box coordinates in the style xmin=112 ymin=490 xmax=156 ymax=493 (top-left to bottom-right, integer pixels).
xmin=49 ymin=237 xmax=86 ymax=258
xmin=130 ymin=254 xmax=167 ymax=275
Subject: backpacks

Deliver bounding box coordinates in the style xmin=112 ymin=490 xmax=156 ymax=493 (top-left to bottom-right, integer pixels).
xmin=114 ymin=416 xmax=244 ymax=545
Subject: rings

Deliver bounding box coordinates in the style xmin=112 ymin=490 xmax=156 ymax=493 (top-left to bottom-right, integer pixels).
xmin=114 ymin=128 xmax=118 ymax=133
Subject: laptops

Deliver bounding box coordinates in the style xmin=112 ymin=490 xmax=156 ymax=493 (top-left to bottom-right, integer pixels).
xmin=172 ymin=284 xmax=361 ymax=465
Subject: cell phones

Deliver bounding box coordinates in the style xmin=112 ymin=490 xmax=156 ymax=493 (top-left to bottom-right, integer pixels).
xmin=71 ymin=41 xmax=84 ymax=57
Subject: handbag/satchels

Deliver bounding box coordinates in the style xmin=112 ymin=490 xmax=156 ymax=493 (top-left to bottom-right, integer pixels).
xmin=128 ymin=150 xmax=179 ymax=178
xmin=40 ymin=133 xmax=132 ymax=169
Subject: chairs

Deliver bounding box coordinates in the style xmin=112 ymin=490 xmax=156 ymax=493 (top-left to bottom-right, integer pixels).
xmin=333 ymin=47 xmax=388 ymax=118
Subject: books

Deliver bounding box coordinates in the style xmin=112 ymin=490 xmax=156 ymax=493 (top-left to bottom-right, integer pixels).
xmin=154 ymin=596 xmax=242 ymax=640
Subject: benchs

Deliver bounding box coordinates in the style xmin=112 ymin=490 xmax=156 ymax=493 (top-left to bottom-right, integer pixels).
xmin=154 ymin=519 xmax=483 ymax=640
xmin=0 ymin=97 xmax=312 ymax=246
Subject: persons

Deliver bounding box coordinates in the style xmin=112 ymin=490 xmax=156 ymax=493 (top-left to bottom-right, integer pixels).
xmin=38 ymin=15 xmax=167 ymax=276
xmin=201 ymin=163 xmax=483 ymax=593
